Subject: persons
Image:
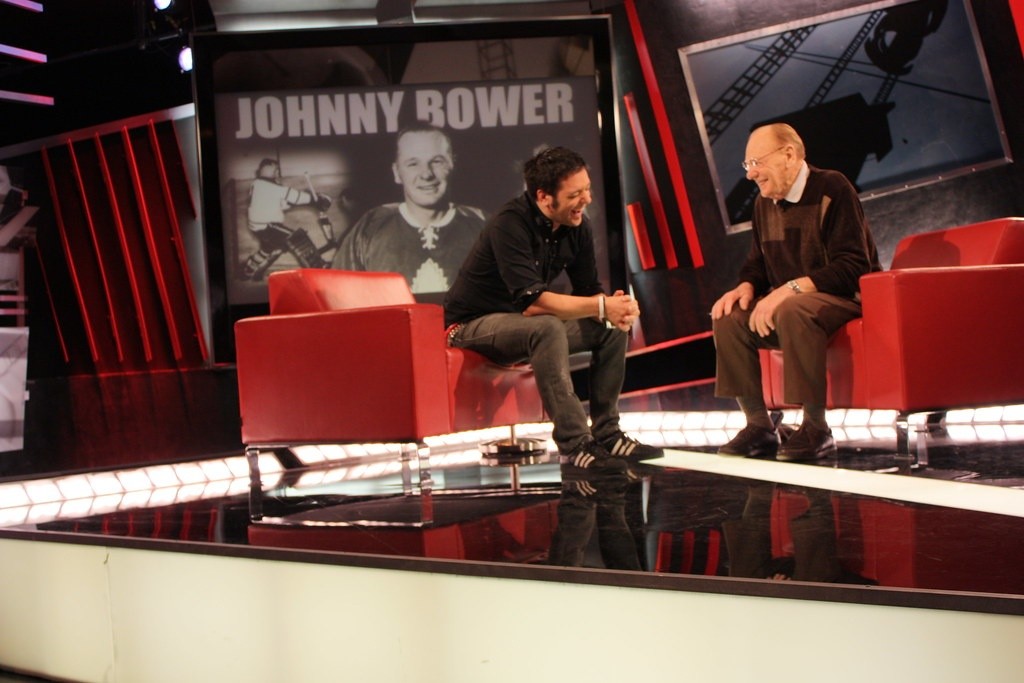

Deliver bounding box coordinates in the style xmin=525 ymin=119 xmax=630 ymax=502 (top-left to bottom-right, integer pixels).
xmin=445 ymin=146 xmax=663 ymax=475
xmin=711 ymin=123 xmax=884 ymax=460
xmin=249 ymin=158 xmax=333 ymax=269
xmin=722 ymin=477 xmax=877 ymax=585
xmin=550 ymin=474 xmax=641 ymax=571
xmin=330 ymin=123 xmax=485 ymax=294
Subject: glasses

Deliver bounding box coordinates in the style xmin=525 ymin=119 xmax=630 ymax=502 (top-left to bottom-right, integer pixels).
xmin=741 ymin=146 xmax=785 ymax=172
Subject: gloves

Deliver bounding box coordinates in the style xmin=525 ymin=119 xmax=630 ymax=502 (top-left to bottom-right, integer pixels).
xmin=310 ymin=192 xmax=332 ymax=211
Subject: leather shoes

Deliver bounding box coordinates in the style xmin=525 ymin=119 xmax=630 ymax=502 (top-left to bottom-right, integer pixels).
xmin=776 ymin=420 xmax=837 ymax=460
xmin=716 ymin=423 xmax=782 ymax=457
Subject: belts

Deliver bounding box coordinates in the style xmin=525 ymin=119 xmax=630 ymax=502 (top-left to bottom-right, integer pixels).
xmin=447 ymin=323 xmax=468 ymax=346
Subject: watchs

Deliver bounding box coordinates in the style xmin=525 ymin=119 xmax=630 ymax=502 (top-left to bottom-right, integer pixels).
xmin=788 ymin=281 xmax=801 ymax=293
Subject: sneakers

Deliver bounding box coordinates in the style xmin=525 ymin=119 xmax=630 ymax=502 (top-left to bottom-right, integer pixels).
xmin=560 ymin=439 xmax=628 ymax=475
xmin=620 ymin=462 xmax=664 ymax=484
xmin=602 ymin=430 xmax=664 ymax=460
xmin=560 ymin=475 xmax=628 ymax=505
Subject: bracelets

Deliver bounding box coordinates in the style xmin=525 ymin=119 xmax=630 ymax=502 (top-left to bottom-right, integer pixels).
xmin=599 ymin=296 xmax=606 ymax=323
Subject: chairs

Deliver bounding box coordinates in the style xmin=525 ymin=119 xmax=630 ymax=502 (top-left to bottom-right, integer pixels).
xmin=759 ymin=216 xmax=1024 ymax=472
xmin=235 ymin=270 xmax=556 ymax=527
